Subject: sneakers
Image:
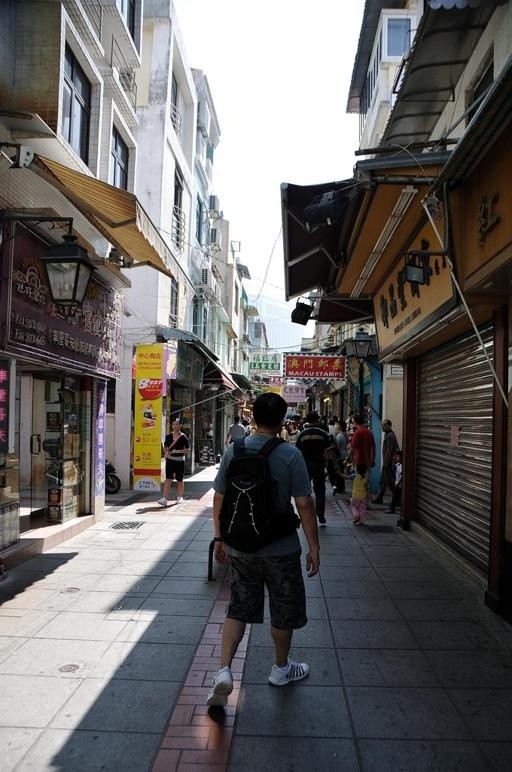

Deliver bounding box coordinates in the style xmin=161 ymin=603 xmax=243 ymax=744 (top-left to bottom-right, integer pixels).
xmin=268 ymin=658 xmax=310 ymax=686
xmin=206 ymin=667 xmax=233 ymax=706
xmin=384 ymin=508 xmax=394 ymax=513
xmin=371 ymin=497 xmax=383 ymax=503
xmin=352 ymin=516 xmax=360 ymax=523
xmin=176 ymin=496 xmax=183 ymax=504
xmin=157 ymin=497 xmax=168 ymax=506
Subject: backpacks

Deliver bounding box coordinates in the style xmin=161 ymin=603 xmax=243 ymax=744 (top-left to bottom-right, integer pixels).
xmin=220 ymin=436 xmax=287 ymax=552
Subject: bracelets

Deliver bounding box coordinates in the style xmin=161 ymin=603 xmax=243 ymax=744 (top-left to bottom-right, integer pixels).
xmin=214 ymin=537 xmax=224 ymax=542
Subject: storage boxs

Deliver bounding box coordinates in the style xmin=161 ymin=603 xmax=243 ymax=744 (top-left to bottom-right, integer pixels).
xmin=47 ymin=487 xmax=81 ymax=524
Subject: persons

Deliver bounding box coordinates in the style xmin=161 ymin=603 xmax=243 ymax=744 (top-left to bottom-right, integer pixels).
xmin=158 ymin=418 xmax=191 ymax=506
xmin=225 ymin=411 xmax=258 ymax=446
xmin=384 ymin=450 xmax=402 ymax=514
xmin=277 ymin=411 xmax=376 ymax=527
xmin=205 ymin=392 xmax=321 ymax=708
xmin=370 ymin=419 xmax=399 ymax=504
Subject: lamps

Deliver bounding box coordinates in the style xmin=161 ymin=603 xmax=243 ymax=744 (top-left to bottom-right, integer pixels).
xmin=203 ymin=382 xmax=220 ymax=392
xmin=291 ymin=297 xmax=315 ymax=325
xmin=324 ymin=394 xmax=333 ymax=403
xmin=1 ymin=213 xmax=99 ymax=319
xmin=0 ymin=142 xmax=35 ymax=169
xmin=105 ymin=248 xmax=124 ymax=266
xmin=403 ymin=251 xmax=447 ymax=284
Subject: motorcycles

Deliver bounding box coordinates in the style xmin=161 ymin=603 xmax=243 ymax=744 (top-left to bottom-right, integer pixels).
xmin=42 ymin=438 xmax=121 ymax=494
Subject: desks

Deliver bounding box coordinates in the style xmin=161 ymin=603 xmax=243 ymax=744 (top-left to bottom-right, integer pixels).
xmin=0 ymin=469 xmax=19 ymax=496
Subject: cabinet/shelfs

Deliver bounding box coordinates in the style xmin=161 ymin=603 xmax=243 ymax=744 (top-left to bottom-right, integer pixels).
xmin=44 ymin=401 xmax=82 ymax=523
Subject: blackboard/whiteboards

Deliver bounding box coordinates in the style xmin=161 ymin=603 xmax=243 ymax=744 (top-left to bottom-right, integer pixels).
xmin=199 ymin=440 xmax=214 ymax=463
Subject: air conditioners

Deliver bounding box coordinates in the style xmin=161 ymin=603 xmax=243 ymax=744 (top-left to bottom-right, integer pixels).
xmin=200 ymin=268 xmax=222 ymax=305
xmin=210 ymin=195 xmax=220 ymax=219
xmin=211 ymin=227 xmax=221 ymax=250
